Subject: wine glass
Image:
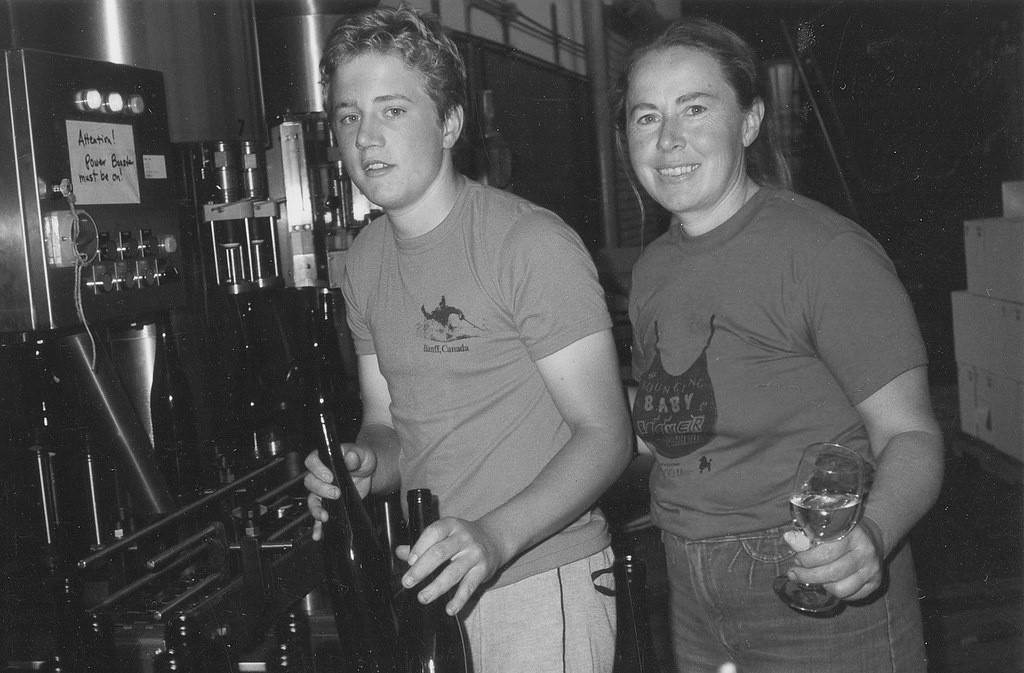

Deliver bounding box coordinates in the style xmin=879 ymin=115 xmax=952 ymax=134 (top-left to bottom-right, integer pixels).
xmin=773 ymin=442 xmax=864 ymax=614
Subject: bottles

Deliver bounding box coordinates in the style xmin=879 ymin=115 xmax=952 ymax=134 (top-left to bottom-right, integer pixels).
xmin=612 ymin=558 xmax=660 ymax=673
xmin=1 ymin=293 xmax=473 ymax=673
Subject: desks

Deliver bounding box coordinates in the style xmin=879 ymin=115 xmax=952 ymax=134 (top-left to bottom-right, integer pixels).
xmin=904 ymin=382 xmax=1024 ymax=673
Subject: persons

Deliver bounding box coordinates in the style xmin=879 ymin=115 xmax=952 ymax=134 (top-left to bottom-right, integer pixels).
xmin=304 ymin=2 xmax=633 ymax=672
xmin=626 ymin=20 xmax=952 ymax=671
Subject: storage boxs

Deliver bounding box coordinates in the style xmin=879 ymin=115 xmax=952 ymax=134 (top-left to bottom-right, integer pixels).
xmin=951 ymin=180 xmax=1023 ymax=461
